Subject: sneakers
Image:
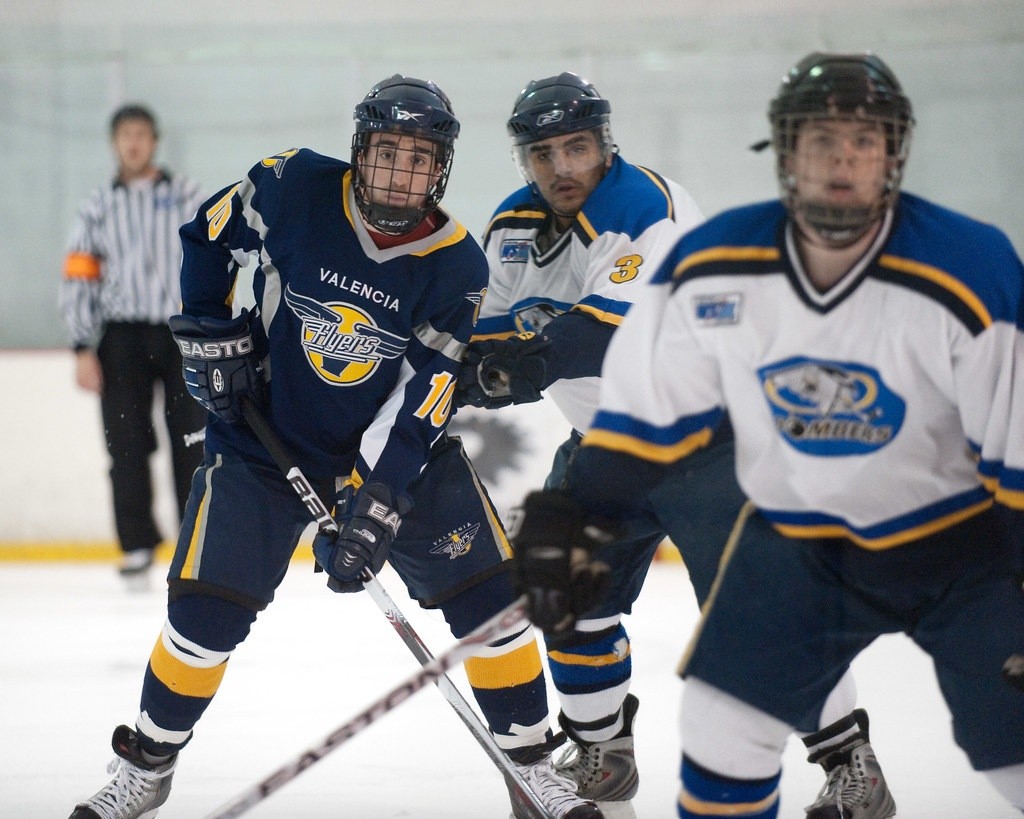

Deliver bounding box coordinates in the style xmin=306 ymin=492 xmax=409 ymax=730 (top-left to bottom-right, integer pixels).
xmin=68 ymin=727 xmax=179 ymax=819
xmin=488 ymin=725 xmax=604 ymax=819
xmin=554 ymin=692 xmax=639 ymax=819
xmin=120 ymin=547 xmax=153 ymax=581
xmin=803 ymin=709 xmax=896 ymax=819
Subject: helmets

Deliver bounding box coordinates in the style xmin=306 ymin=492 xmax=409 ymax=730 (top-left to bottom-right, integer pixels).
xmin=506 ymin=71 xmax=611 ymax=144
xmin=768 ymin=53 xmax=913 ymax=178
xmin=349 ymin=75 xmax=460 ymax=169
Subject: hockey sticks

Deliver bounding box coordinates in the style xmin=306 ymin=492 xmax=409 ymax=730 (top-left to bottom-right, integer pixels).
xmin=203 ymin=594 xmax=531 ymax=819
xmin=235 ymin=392 xmax=557 ymax=819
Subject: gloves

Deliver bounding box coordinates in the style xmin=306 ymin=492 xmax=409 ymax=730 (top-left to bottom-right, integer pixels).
xmin=452 ymin=331 xmax=542 ymax=410
xmin=510 ymin=491 xmax=616 ymax=636
xmin=312 ymin=482 xmax=404 ymax=582
xmin=168 ymin=308 xmax=266 ymax=425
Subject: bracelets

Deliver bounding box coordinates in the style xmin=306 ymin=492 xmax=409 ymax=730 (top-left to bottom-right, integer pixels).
xmin=72 ymin=344 xmax=92 ymax=355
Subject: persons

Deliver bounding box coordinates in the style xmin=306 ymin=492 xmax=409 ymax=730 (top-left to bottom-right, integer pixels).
xmin=446 ymin=52 xmax=1024 ymax=819
xmin=66 ymin=75 xmax=604 ymax=819
